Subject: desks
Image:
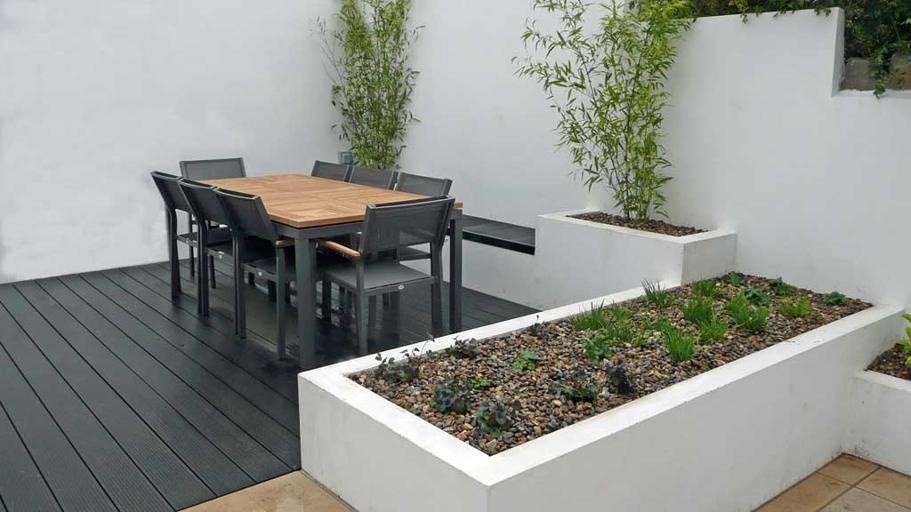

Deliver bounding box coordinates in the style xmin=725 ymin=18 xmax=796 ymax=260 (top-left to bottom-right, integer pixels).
xmin=190 ymin=172 xmax=450 ymax=370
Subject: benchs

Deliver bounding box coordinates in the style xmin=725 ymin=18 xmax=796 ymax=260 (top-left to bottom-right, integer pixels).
xmin=448 ymin=216 xmax=535 ymax=254
xmin=212 ymin=187 xmax=296 ymax=361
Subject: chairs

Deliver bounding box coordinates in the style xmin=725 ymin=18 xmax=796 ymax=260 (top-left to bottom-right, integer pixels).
xmin=180 ymin=157 xmax=247 ymax=289
xmin=151 ymin=171 xmax=232 ymax=306
xmin=177 ymin=178 xmax=274 ymax=335
xmin=311 ymin=160 xmax=352 ymax=182
xmin=349 ymin=165 xmax=398 ymax=190
xmin=384 ymin=172 xmax=453 ymax=261
xmin=320 ymin=196 xmax=457 ymax=355
